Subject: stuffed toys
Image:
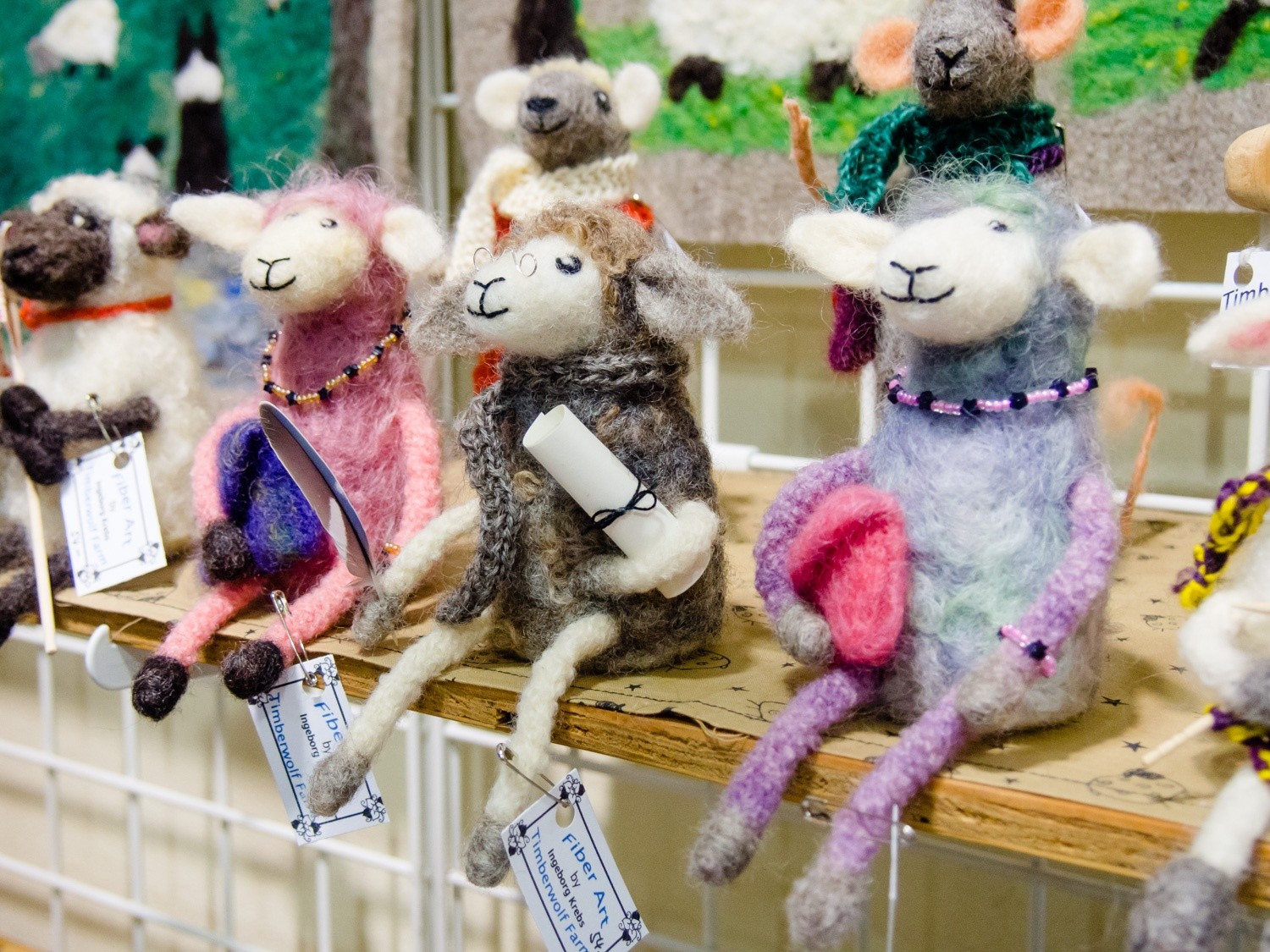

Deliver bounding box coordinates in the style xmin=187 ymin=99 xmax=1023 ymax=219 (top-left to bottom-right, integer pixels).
xmin=690 ymin=169 xmax=1163 ymax=943
xmin=1131 ymin=120 xmax=1270 ymax=950
xmin=306 ymin=198 xmax=753 ymax=890
xmin=781 ymin=0 xmax=1068 ymax=376
xmin=0 ymin=172 xmax=216 ymax=655
xmin=132 ymin=155 xmax=445 ymax=722
xmin=448 ymin=51 xmax=753 ymax=393
